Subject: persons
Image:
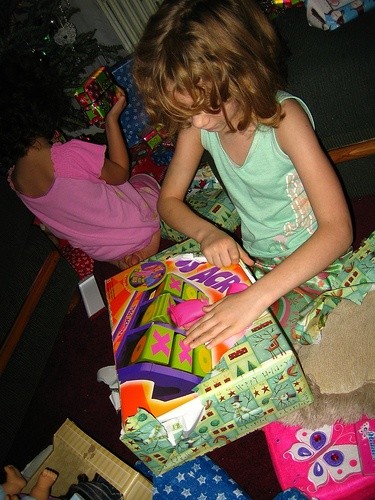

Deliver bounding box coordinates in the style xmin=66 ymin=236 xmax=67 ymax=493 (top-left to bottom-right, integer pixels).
xmin=132 ymin=0 xmax=375 ymax=351
xmin=0 ymin=465 xmax=57 ymax=500
xmin=0 ymin=53 xmax=165 ymax=271
